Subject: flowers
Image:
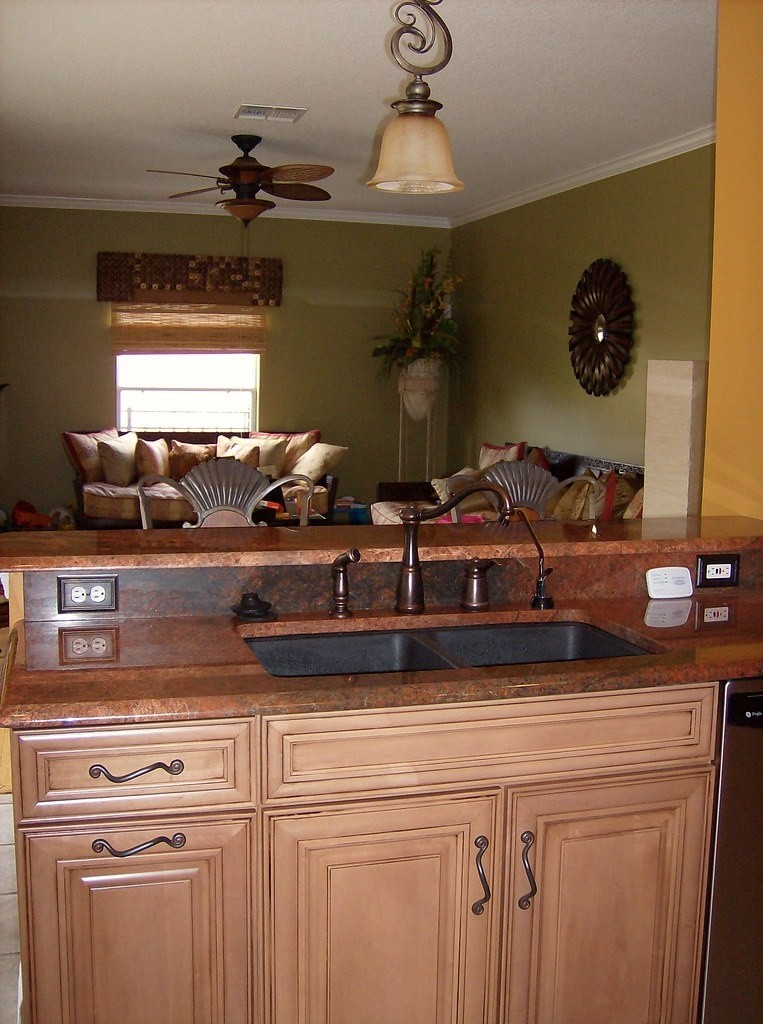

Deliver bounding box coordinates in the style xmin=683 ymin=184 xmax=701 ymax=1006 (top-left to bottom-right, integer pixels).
xmin=371 ymin=242 xmax=477 ymax=372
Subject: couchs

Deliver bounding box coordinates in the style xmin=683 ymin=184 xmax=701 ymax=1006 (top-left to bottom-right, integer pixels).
xmin=370 ymin=442 xmax=644 ymax=525
xmin=71 ymin=430 xmax=339 ymax=530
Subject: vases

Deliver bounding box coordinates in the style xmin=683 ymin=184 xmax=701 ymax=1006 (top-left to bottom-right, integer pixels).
xmin=399 ymin=358 xmax=442 ymax=422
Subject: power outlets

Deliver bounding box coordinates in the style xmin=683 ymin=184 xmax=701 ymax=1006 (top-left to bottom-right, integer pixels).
xmin=694 ymin=596 xmax=737 ymax=630
xmin=57 ymin=574 xmax=119 ymax=612
xmin=57 ymin=626 xmax=119 ymax=667
xmin=696 ymin=554 xmax=740 ymax=587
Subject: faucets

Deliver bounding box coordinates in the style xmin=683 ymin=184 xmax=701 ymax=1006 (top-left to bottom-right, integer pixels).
xmin=497 ymin=496 xmax=556 ymax=611
xmin=390 ymin=481 xmax=514 ymax=616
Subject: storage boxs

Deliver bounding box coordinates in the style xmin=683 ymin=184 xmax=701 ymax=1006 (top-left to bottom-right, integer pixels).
xmin=332 ymin=503 xmax=369 ymax=526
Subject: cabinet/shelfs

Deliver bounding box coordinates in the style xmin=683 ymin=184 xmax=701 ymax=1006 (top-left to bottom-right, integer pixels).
xmin=10 ymin=716 xmax=259 ymax=1024
xmin=260 ymin=681 xmax=720 ymax=1024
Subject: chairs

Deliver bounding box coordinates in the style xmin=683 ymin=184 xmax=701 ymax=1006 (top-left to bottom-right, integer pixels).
xmin=136 ymin=458 xmax=315 ymax=530
xmin=446 ymin=461 xmax=600 ymax=524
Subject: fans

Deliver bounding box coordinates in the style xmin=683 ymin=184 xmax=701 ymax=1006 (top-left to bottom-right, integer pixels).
xmin=145 ymin=134 xmax=334 ymax=202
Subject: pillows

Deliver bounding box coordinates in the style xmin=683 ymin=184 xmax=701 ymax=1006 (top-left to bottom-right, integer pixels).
xmin=524 ymin=448 xmax=549 ymax=471
xmin=288 ymin=442 xmax=348 ymax=488
xmin=98 ymin=432 xmax=138 ymax=487
xmin=216 ymin=435 xmax=260 ymax=470
xmin=431 ymin=466 xmax=493 ymax=512
xmin=544 ymin=467 xmax=643 ymax=520
xmin=134 ymin=438 xmax=169 ymax=486
xmin=62 ymin=427 xmax=119 ymax=482
xmin=230 ymin=436 xmax=287 ymax=480
xmin=248 ymin=430 xmax=321 ymax=477
xmin=478 ymin=441 xmax=525 ymax=469
xmin=169 ymin=439 xmax=217 ymax=479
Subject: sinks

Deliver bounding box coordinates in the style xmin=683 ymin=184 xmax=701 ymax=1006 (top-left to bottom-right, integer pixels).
xmin=242 ymin=631 xmax=459 ymax=680
xmin=426 ymin=623 xmax=657 ymax=669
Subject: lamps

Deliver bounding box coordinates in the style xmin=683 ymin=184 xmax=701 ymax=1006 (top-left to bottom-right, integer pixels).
xmin=366 ymin=0 xmax=464 ymax=194
xmin=215 ymin=198 xmax=277 ymax=230
